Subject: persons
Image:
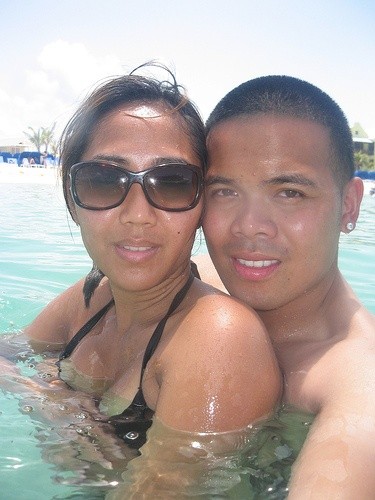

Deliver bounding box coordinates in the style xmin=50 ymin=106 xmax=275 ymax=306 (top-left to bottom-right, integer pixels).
xmin=33 ymin=75 xmax=375 ymax=500
xmin=0 ymin=61 xmax=283 ymax=500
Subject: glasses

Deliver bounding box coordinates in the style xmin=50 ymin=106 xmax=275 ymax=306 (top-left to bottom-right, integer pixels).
xmin=65 ymin=162 xmax=206 ymax=214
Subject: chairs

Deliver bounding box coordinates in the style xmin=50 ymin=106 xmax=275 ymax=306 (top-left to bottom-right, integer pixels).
xmin=0 ymin=151 xmax=61 ymax=169
